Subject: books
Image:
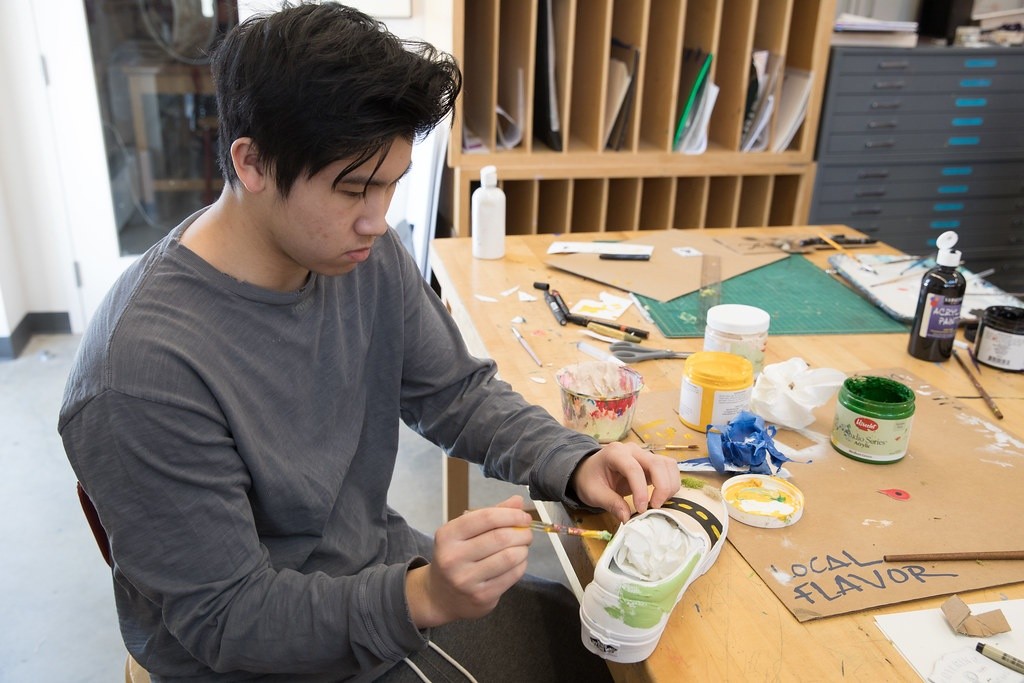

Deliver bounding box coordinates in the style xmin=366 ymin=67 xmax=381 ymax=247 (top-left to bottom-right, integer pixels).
xmin=831 ymin=14 xmax=918 ymax=47
xmin=603 ymin=38 xmax=813 ymax=152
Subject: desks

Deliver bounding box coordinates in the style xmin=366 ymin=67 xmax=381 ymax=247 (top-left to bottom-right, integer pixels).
xmin=106 ymin=22 xmax=226 ymax=207
xmin=423 ymin=223 xmax=1024 ymax=682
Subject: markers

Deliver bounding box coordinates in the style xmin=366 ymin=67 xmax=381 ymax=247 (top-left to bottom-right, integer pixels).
xmin=543 ymin=289 xmax=571 ymax=326
xmin=566 ymin=314 xmax=650 ymax=339
xmin=975 ymin=641 xmax=1024 ymax=675
xmin=800 ymin=233 xmax=877 ymax=245
xmin=598 ymin=253 xmax=651 ymax=261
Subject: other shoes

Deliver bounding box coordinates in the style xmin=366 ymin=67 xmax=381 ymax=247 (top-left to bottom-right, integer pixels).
xmin=575 ymin=479 xmax=730 ymax=663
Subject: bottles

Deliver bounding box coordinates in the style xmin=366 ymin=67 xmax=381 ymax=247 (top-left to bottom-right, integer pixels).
xmin=907 ymin=231 xmax=967 ymax=363
xmin=471 ymin=163 xmax=507 ymax=259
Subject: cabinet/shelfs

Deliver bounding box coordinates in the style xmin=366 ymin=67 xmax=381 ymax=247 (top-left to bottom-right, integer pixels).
xmin=444 ymin=1 xmax=837 ymax=236
xmin=806 ymin=29 xmax=1024 ymax=304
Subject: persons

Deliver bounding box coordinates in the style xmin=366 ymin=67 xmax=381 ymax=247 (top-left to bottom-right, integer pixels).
xmin=56 ymin=1 xmax=680 ymax=683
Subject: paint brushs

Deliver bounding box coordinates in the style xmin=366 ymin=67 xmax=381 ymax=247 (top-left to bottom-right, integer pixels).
xmin=871 ymin=252 xmax=933 ymax=288
xmin=511 ymin=326 xmax=541 ymax=367
xmin=661 ymin=443 xmax=700 ymax=452
xmin=810 ymin=226 xmax=879 ymax=277
xmin=462 ymin=505 xmax=615 ymax=543
xmin=951 ymin=349 xmax=1003 ymax=421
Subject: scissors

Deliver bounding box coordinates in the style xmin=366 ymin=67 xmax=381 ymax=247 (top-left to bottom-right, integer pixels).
xmin=610 ymin=341 xmax=695 ymax=364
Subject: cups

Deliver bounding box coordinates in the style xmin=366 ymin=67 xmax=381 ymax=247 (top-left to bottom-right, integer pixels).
xmin=555 ymin=361 xmax=644 ymax=445
xmin=830 ymin=375 xmax=916 ymax=465
xmin=703 ymin=304 xmax=771 ymax=381
xmin=972 ymin=305 xmax=1024 ymax=374
xmin=678 ymin=351 xmax=754 ymax=434
